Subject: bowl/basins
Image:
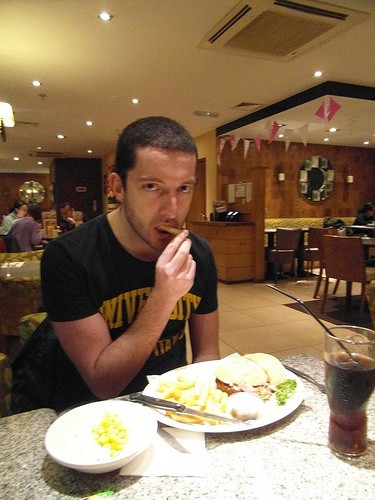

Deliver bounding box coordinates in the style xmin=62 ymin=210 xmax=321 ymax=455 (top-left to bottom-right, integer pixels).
xmin=44 ymin=400 xmax=158 ymax=474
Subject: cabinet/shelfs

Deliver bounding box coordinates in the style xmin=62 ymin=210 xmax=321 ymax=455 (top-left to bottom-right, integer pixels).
xmin=190 ymin=220 xmax=258 ymax=283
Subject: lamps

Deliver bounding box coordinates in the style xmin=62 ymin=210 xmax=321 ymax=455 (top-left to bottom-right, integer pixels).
xmin=0 ymin=102 xmax=15 ymax=142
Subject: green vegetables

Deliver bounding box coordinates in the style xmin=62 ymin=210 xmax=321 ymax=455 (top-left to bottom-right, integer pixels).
xmin=275 ymin=378 xmax=297 ymax=405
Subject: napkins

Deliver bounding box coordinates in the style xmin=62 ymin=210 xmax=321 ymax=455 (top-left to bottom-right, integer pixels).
xmin=119 ymin=426 xmax=212 ymax=477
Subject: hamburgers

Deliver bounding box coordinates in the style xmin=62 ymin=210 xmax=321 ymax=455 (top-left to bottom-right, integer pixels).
xmin=216 ymin=351 xmax=286 ymax=401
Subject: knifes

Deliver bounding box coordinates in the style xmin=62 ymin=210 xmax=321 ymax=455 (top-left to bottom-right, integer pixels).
xmin=130 ymin=392 xmax=241 ymax=423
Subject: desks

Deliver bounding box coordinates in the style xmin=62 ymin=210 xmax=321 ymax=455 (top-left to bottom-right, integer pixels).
xmin=343 ymin=225 xmax=375 ymax=236
xmin=264 ymin=228 xmax=309 ymax=247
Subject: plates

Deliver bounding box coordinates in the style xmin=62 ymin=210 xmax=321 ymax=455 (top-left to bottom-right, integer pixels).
xmin=141 ymin=359 xmax=306 ymax=434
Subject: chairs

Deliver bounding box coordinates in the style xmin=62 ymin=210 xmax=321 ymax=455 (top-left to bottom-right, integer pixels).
xmin=321 ymin=234 xmax=375 ymax=317
xmin=304 ymin=227 xmax=338 ymax=275
xmin=270 ymin=229 xmax=301 ymax=284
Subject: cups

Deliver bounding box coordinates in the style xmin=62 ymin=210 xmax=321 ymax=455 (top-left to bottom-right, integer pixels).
xmin=47 ymin=225 xmax=55 ymax=238
xmin=57 ymin=230 xmax=62 ymax=238
xmin=322 ymin=326 xmax=375 ymax=461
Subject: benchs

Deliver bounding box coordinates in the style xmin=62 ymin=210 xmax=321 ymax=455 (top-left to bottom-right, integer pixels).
xmin=264 ymin=216 xmax=357 ymax=264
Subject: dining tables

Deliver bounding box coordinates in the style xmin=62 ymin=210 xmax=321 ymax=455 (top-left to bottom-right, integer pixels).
xmin=0 ymin=351 xmax=375 ymax=500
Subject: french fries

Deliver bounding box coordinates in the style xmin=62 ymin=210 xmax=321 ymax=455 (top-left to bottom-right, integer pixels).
xmin=92 ymin=414 xmax=128 ymax=452
xmin=158 ymin=225 xmax=182 ymax=235
xmin=157 ymin=375 xmax=230 ymax=427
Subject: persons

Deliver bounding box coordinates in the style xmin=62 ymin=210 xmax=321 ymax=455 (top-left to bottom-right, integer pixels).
xmin=10 ymin=116 xmax=220 ymax=415
xmin=353 ymin=204 xmax=375 ymax=225
xmin=1 ymin=199 xmax=42 ymax=252
xmin=59 ymin=202 xmax=88 ymax=234
